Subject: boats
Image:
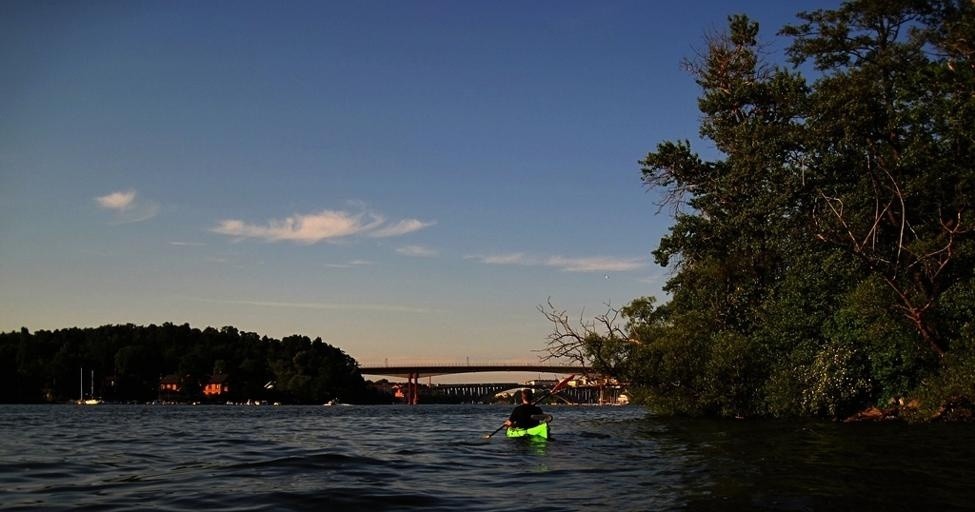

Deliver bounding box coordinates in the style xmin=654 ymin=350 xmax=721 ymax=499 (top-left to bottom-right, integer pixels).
xmin=505 ymin=421 xmax=550 ymax=440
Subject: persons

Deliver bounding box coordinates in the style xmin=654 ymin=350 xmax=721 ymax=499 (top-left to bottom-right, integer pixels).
xmin=503 ymin=389 xmax=545 ymax=429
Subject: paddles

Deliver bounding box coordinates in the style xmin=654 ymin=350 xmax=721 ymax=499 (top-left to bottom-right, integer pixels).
xmin=485 ymin=375 xmax=576 ymax=440
